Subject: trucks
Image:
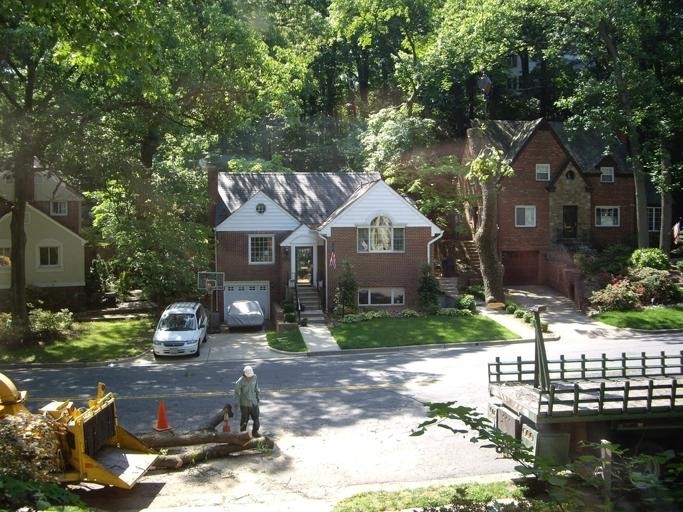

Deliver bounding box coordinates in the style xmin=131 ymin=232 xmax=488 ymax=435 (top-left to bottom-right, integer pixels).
xmin=486 ymin=304 xmax=683 ymax=494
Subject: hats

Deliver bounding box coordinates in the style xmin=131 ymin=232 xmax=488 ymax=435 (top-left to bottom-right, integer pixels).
xmin=243 ymin=365 xmax=254 ymax=378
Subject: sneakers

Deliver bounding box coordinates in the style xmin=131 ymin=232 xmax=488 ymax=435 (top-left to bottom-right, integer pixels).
xmin=252 ymin=432 xmax=261 ymax=437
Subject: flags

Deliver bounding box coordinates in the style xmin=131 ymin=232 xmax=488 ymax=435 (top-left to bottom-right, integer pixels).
xmin=327 ymin=244 xmax=336 ymax=271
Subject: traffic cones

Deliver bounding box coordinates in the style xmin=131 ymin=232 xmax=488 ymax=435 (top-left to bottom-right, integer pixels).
xmin=152 ymin=399 xmax=174 ymax=432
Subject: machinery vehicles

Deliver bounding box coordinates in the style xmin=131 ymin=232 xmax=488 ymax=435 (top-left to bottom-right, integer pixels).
xmin=0 ymin=373 xmax=159 ymax=490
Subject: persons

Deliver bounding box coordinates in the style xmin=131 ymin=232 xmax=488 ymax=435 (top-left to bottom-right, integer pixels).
xmin=231 ymin=365 xmax=262 ymax=438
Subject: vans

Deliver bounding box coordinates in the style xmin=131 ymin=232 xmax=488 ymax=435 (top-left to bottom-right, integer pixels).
xmin=153 ymin=301 xmax=208 ymax=359
